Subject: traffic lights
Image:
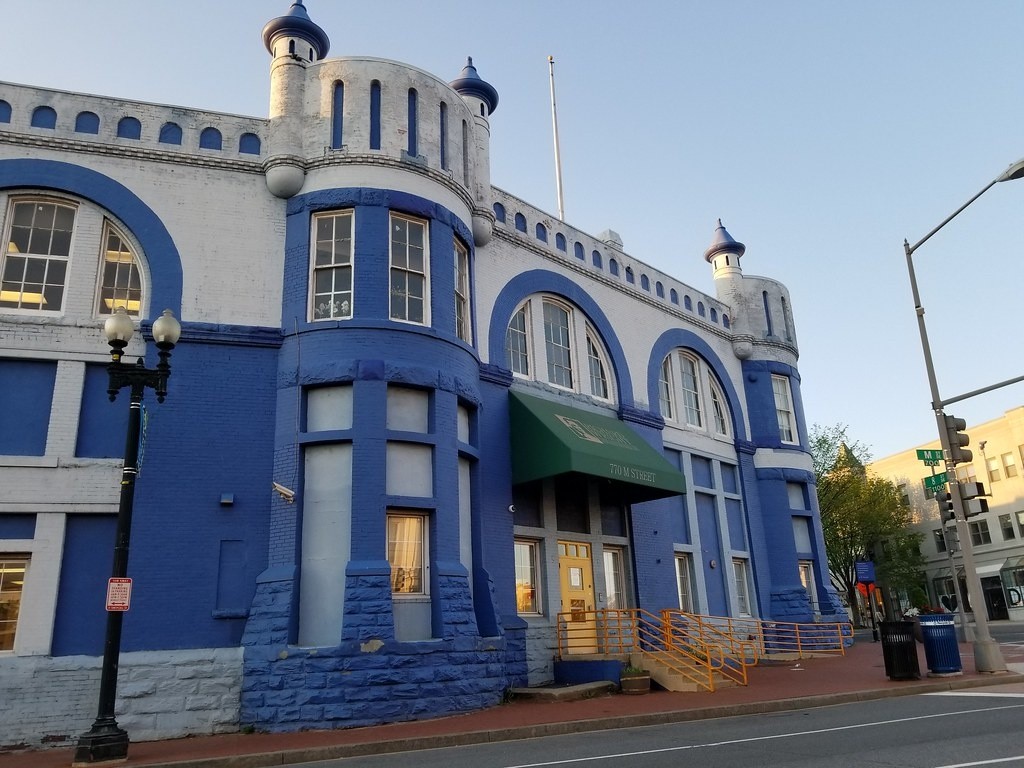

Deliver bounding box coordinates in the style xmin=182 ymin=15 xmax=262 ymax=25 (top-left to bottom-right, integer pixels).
xmin=945 ymin=415 xmax=972 ymax=466
xmin=935 ymin=492 xmax=955 ymax=524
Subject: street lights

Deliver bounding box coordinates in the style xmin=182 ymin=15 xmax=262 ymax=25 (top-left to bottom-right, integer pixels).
xmin=70 ymin=302 xmax=182 ymax=766
xmin=902 ymin=160 xmax=1024 ymax=674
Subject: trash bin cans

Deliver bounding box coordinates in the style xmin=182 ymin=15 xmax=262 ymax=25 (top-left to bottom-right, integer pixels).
xmin=878 ymin=620 xmax=921 ymax=679
xmin=917 ymin=612 xmax=963 ymax=674
